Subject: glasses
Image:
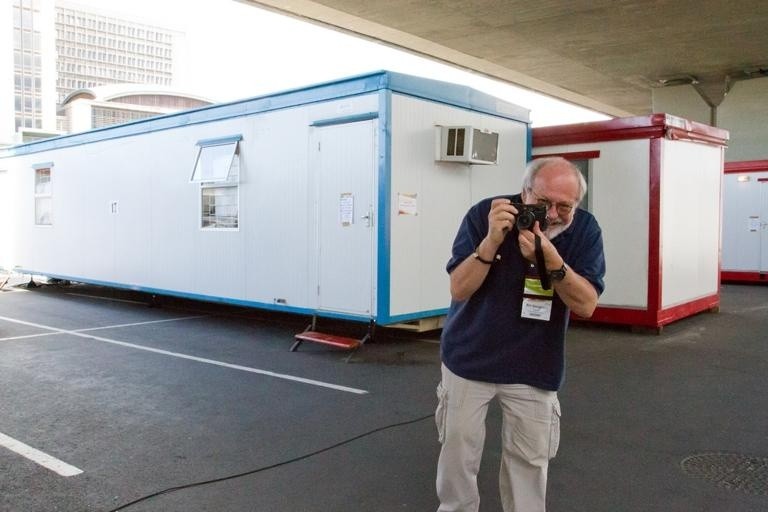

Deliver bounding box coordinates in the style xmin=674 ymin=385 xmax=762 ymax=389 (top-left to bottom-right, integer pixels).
xmin=532 ymin=189 xmax=573 ymax=215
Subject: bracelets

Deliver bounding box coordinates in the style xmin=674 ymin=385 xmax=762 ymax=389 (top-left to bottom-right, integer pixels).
xmin=472 ymin=240 xmax=500 ymax=265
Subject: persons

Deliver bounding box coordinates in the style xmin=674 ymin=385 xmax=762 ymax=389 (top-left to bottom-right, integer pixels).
xmin=434 ymin=157 xmax=607 ymax=511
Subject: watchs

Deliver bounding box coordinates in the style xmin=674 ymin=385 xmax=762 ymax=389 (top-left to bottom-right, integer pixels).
xmin=542 ymin=261 xmax=568 ymax=283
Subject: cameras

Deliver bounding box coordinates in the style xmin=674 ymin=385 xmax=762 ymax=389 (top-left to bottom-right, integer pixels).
xmin=509 ymin=203 xmax=547 ymax=231
